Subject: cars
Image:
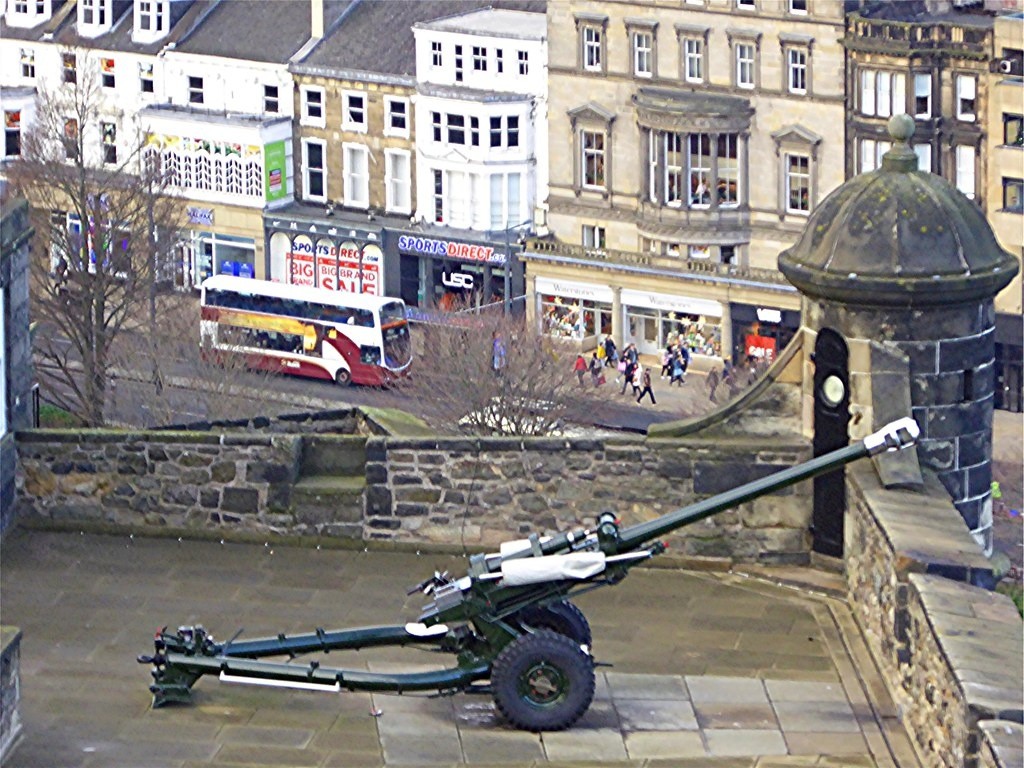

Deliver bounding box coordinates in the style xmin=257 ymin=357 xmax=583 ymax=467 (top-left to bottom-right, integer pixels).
xmin=457 ymin=398 xmax=569 ymax=435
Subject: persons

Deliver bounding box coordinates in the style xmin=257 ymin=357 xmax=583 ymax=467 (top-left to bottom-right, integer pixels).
xmin=589 ymin=334 xmax=643 ymax=397
xmin=110 ymin=372 xmax=117 ymax=392
xmin=246 ymin=331 xmax=303 ymax=353
xmin=210 ymin=291 xmax=373 ymax=325
xmin=705 ymin=354 xmax=760 ymax=402
xmin=661 ymin=336 xmax=692 ymax=387
xmin=636 ymin=368 xmax=658 ymax=406
xmin=153 ymin=365 xmax=166 ymax=396
xmin=573 ymin=355 xmax=587 ymax=388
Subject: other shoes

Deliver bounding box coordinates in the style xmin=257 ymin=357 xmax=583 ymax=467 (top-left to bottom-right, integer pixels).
xmin=652 ymin=402 xmax=659 ymax=407
xmin=616 ymin=379 xmax=619 ymax=383
xmin=684 ymin=372 xmax=688 ymax=376
xmin=631 ymin=393 xmax=636 ymax=396
xmin=619 ymin=392 xmax=624 ymax=395
xmin=661 ymin=376 xmax=665 ymax=380
xmin=667 ymin=376 xmax=671 ymax=379
xmin=636 ymin=402 xmax=641 ymax=406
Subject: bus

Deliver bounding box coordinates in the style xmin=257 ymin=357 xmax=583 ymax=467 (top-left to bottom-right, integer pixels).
xmin=194 ymin=276 xmax=413 ymax=390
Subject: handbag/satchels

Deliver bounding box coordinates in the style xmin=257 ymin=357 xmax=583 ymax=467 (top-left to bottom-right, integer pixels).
xmin=598 ymin=376 xmax=606 ymax=385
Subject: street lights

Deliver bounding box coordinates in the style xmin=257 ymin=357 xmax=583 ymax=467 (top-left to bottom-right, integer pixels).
xmin=500 ymin=216 xmax=535 ymax=320
xmin=147 ymin=169 xmax=180 ymax=374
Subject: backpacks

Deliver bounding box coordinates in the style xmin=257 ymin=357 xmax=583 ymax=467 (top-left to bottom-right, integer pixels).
xmin=593 ymin=358 xmax=601 ymax=369
xmin=611 ymin=349 xmax=618 ymax=360
